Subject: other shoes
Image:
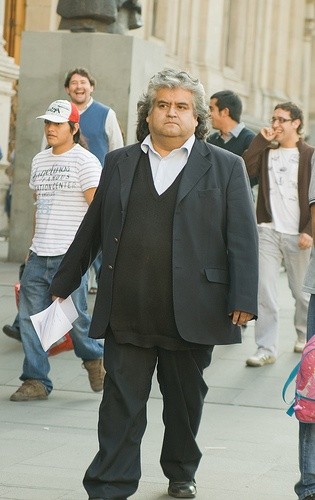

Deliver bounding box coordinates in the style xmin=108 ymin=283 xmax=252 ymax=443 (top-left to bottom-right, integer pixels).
xmin=3 ymin=324 xmax=20 ymax=340
xmin=89 ymin=287 xmax=97 ymax=294
xmin=81 ymin=359 xmax=105 ymax=392
xmin=10 ymin=379 xmax=48 ymax=401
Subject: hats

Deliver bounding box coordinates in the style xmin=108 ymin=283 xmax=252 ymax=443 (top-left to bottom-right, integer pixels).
xmin=35 ymin=100 xmax=80 ymax=123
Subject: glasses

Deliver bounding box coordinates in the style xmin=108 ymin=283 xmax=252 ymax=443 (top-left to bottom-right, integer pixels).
xmin=271 ymin=118 xmax=293 ymax=124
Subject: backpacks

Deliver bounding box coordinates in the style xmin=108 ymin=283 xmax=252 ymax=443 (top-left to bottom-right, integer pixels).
xmin=282 ymin=336 xmax=315 ymax=423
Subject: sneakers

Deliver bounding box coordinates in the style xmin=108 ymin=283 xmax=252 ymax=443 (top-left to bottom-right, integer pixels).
xmin=245 ymin=352 xmax=274 ymax=367
xmin=294 ymin=342 xmax=306 ymax=352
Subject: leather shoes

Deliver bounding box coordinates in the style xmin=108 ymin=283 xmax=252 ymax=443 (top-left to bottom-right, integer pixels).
xmin=168 ymin=479 xmax=197 ymax=498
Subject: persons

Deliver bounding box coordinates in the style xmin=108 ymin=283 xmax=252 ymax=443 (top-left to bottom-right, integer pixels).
xmin=243 ymin=101 xmax=315 ymax=368
xmin=206 ymin=88 xmax=261 ymax=211
xmin=46 ymin=67 xmax=261 ymax=500
xmin=1 ymin=310 xmax=24 ymax=342
xmin=293 ymin=147 xmax=315 ymax=500
xmin=7 ymin=98 xmax=108 ymax=404
xmin=2 ymin=65 xmax=127 ymax=296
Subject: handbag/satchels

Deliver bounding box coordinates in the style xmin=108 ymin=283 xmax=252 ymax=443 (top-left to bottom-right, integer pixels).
xmin=15 ymin=284 xmax=74 ymax=356
